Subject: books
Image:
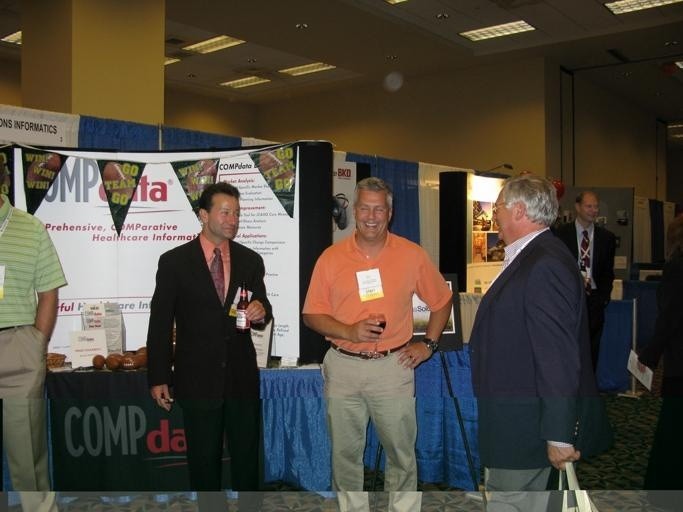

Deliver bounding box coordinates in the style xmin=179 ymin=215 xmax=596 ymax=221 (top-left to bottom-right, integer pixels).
xmin=627 ymin=349 xmax=654 ymax=392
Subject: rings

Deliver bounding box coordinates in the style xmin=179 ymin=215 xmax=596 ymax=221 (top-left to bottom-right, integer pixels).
xmin=413 ymin=358 xmax=416 ymax=362
xmin=408 ymin=352 xmax=412 ymax=358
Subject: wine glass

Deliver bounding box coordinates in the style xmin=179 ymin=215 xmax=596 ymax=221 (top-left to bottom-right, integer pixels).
xmin=366 ymin=312 xmax=386 ymax=359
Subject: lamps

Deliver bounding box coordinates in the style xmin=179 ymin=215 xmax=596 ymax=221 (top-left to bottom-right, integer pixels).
xmin=478 ymin=163 xmax=513 ymax=175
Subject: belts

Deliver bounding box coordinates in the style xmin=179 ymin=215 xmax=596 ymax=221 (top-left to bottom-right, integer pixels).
xmin=329 ymin=338 xmax=412 ymax=360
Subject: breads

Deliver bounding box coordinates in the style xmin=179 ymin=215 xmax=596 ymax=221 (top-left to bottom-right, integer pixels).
xmin=103 ymin=161 xmax=130 ymax=199
xmin=260 ymin=151 xmax=295 ymax=183
xmin=187 ymin=160 xmax=217 ymax=196
xmin=93 ymin=347 xmax=148 ymax=369
xmin=26 ymin=153 xmax=60 ymax=185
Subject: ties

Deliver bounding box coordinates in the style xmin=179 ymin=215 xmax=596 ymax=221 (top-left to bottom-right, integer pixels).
xmin=209 ymin=248 xmax=225 ymax=305
xmin=579 ymin=230 xmax=591 ymax=289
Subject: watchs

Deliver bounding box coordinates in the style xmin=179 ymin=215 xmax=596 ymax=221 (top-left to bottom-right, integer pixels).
xmin=423 ymin=338 xmax=439 ymax=352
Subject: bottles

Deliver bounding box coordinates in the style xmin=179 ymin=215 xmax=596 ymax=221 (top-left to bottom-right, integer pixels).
xmin=578 ymin=257 xmax=586 ymax=290
xmin=473 ymin=279 xmax=481 ymax=293
xmin=235 ymin=282 xmax=250 ymax=334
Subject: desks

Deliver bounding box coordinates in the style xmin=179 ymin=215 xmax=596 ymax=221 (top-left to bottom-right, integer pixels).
xmin=47 ymin=348 xmax=480 ymax=494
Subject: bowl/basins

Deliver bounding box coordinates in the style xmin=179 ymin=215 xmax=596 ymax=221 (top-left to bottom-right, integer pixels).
xmin=46 ymin=353 xmax=66 ymax=368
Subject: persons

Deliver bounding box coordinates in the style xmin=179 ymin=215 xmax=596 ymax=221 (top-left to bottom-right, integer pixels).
xmin=0 ymin=154 xmax=68 ymax=512
xmin=468 ymin=171 xmax=615 ymax=512
xmin=146 ymin=183 xmax=273 ymax=512
xmin=637 ymin=215 xmax=683 ymax=512
xmin=557 ymin=191 xmax=616 ymax=374
xmin=302 ymin=177 xmax=454 ymax=512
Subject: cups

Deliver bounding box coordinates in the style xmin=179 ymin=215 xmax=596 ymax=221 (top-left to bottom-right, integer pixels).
xmin=481 ymin=280 xmax=491 ymax=293
